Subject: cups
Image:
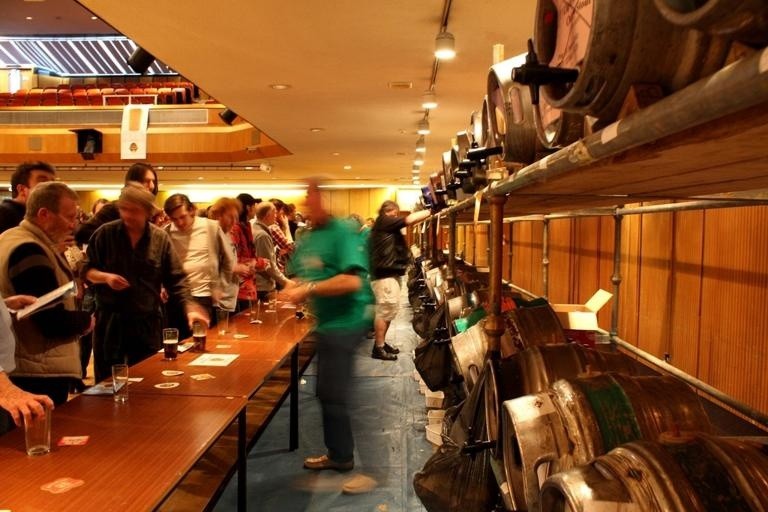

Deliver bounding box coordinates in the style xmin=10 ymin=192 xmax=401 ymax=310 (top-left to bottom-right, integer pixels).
xmin=268 ymin=289 xmax=277 ymax=310
xmin=249 ymin=298 xmax=260 ymax=323
xmin=217 ymin=308 xmax=229 ymax=333
xmin=112 ymin=365 xmax=130 ymax=403
xmin=192 ymin=323 xmax=207 ymax=353
xmin=23 ymin=406 xmax=50 ymax=457
xmin=162 ymin=328 xmax=179 ymax=360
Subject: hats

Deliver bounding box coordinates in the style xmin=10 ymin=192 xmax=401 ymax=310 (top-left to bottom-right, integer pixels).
xmin=237 ymin=192 xmax=262 ymax=205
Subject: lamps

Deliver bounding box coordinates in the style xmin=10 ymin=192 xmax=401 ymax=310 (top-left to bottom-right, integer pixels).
xmin=80 ymin=137 xmax=97 ymax=160
xmin=411 ymin=27 xmax=461 ymax=188
xmin=127 ymin=46 xmax=154 ymax=75
xmin=217 ymin=104 xmax=237 ymax=127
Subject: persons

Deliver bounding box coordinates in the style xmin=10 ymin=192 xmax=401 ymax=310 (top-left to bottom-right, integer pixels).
xmin=0 ymin=160 xmax=55 ymax=234
xmin=1 ymin=291 xmax=55 ymax=434
xmin=0 ymin=181 xmax=96 ymax=407
xmin=75 ymin=163 xmax=434 ymax=496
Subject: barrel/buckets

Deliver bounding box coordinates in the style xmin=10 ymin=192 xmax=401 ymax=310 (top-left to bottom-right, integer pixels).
xmin=414 ymin=0 xmax=768 ymax=215
xmin=407 ymin=241 xmax=768 ymax=512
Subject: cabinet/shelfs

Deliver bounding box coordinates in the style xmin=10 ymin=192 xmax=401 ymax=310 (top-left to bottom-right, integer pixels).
xmin=406 ymin=43 xmax=768 ymax=510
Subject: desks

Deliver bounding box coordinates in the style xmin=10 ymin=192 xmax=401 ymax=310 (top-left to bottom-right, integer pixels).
xmin=194 ymin=295 xmax=320 ymax=399
xmin=0 ymin=393 xmax=249 ymax=511
xmin=78 ymin=337 xmax=301 ymax=511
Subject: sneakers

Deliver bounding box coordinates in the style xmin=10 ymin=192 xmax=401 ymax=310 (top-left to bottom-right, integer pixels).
xmin=371 ymin=348 xmax=397 ymax=361
xmin=372 ymin=342 xmax=399 ymax=354
xmin=343 ymin=475 xmax=379 ymax=495
xmin=304 ymin=454 xmax=353 ymax=472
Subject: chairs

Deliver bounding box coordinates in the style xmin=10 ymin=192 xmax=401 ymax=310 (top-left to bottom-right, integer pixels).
xmin=0 ymin=80 xmax=201 ymax=107
